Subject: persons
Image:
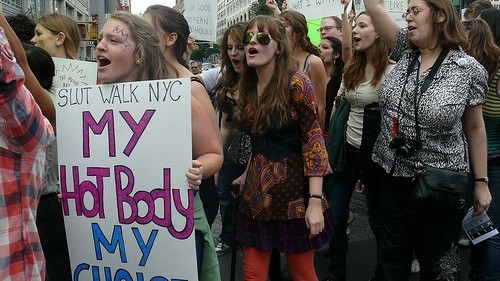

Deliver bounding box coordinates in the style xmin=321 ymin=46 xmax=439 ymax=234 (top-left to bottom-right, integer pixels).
xmin=56 ymin=9 xmax=224 ymax=281
xmin=229 ymin=14 xmax=334 ymax=281
xmin=0 ymin=27 xmax=55 ymax=280
xmin=364 ymin=0 xmax=492 ymax=281
xmin=0 ymin=0 xmax=400 ymax=281
xmin=459 ymin=1 xmax=500 ymax=280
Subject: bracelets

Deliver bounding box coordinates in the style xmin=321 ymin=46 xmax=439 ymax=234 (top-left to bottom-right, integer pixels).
xmin=308 ymin=194 xmax=322 ymax=199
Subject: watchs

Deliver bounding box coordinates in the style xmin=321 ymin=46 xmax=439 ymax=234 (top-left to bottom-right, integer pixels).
xmin=474 ymin=177 xmax=488 ymax=182
